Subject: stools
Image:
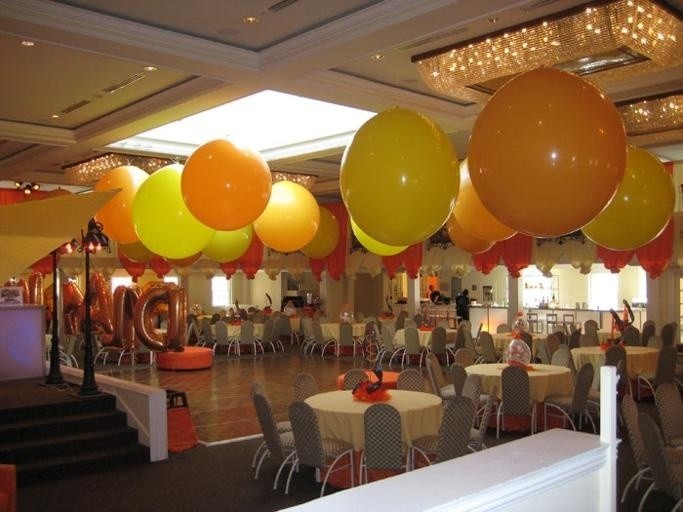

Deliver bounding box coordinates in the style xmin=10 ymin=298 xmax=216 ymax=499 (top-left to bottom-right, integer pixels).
xmin=160 ymin=346 xmax=212 ymax=370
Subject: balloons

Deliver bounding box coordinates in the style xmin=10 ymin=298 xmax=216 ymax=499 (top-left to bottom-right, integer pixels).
xmin=203 ymin=223 xmax=253 ymax=262
xmin=339 ymin=107 xmax=460 ymax=246
xmin=93 ymin=165 xmax=150 ymax=246
xmin=349 ymin=215 xmax=410 ymax=257
xmin=446 ymin=212 xmax=496 ymax=256
xmin=180 ymin=138 xmax=274 ymax=233
xmin=464 ymin=63 xmax=628 ymax=240
xmin=163 ymin=251 xmax=202 ymax=267
xmin=118 ymin=240 xmax=155 ymax=264
xmin=454 ymin=156 xmax=518 ymax=242
xmin=132 ymin=163 xmax=215 ymax=260
xmin=580 ymin=142 xmax=678 ymax=253
xmin=299 ymin=204 xmax=340 ymax=259
xmin=252 ymin=178 xmax=321 ymax=253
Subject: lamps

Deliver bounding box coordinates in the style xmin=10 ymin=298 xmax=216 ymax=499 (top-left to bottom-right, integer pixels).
xmin=270 ymin=170 xmax=319 ymax=192
xmin=46 ymin=239 xmax=78 ymax=384
xmin=15 ymin=181 xmax=41 ymax=196
xmin=77 ymin=218 xmax=110 ymax=395
xmin=61 ymin=152 xmax=187 ymax=192
xmin=616 ymin=95 xmax=683 ymax=137
xmin=412 ymin=0 xmax=682 ymax=104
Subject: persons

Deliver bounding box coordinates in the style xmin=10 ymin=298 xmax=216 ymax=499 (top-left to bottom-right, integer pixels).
xmin=455 ymin=289 xmax=470 ymax=326
xmin=428 ymin=284 xmax=443 ymax=306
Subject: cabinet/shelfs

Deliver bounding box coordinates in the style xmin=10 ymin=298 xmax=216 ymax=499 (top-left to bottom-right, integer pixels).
xmin=0 ymin=304 xmax=45 ymax=381
